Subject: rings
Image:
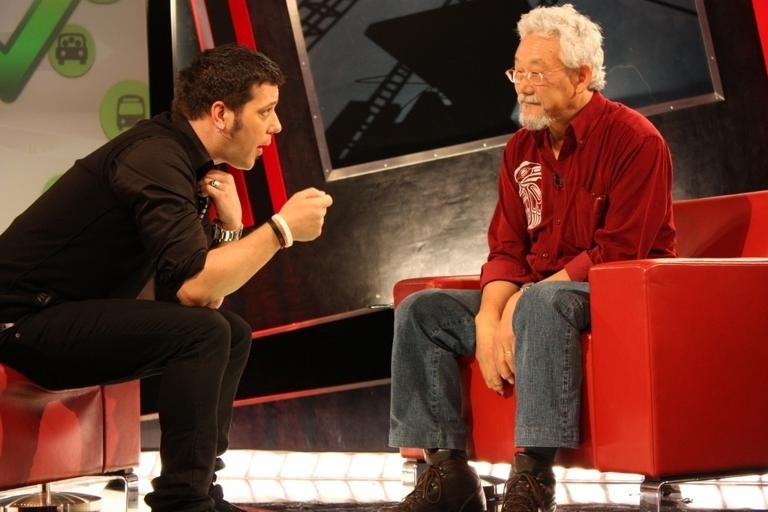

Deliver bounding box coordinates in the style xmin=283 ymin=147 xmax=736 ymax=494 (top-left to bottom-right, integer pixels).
xmin=504 ymin=351 xmax=511 ymax=354
xmin=210 ymin=180 xmax=220 ymax=188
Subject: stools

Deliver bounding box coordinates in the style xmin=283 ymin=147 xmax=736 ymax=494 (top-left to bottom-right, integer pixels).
xmin=0 ymin=359 xmax=143 ymax=511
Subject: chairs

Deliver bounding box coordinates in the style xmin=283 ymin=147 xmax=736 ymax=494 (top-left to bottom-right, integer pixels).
xmin=391 ymin=189 xmax=768 ymax=510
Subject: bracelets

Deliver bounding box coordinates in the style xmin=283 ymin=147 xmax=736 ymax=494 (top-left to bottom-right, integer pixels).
xmin=267 ymin=218 xmax=286 ymax=250
xmin=271 ymin=214 xmax=293 ymax=249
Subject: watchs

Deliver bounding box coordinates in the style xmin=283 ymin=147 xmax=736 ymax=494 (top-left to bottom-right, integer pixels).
xmin=520 ymin=282 xmax=535 ymax=292
xmin=212 ymin=224 xmax=244 ymax=243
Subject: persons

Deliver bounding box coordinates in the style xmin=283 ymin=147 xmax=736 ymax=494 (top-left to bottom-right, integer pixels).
xmin=0 ymin=42 xmax=333 ymax=512
xmin=376 ymin=3 xmax=676 ymax=512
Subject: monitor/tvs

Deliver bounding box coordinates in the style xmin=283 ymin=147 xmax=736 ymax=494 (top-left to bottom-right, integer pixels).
xmin=0 ymin=1 xmax=174 ymax=236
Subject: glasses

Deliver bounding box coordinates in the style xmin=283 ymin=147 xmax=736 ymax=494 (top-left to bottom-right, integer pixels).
xmin=505 ymin=66 xmax=566 ymax=85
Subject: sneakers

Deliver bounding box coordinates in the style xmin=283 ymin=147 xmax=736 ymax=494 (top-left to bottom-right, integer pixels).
xmin=215 ymin=499 xmax=242 ymax=511
xmin=500 ymin=452 xmax=554 ymax=512
xmin=377 ymin=449 xmax=488 ymax=511
